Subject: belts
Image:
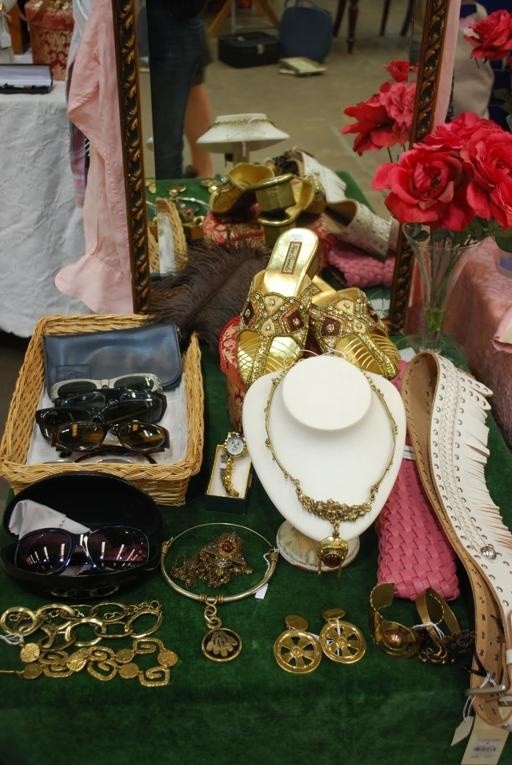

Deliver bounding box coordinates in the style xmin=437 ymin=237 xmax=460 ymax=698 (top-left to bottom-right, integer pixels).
xmin=281 ymin=144 xmax=393 ymax=261
xmin=400 ymin=344 xmax=512 ymax=730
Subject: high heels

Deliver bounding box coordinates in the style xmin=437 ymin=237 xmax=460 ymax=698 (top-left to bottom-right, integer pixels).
xmin=254 ymin=172 xmax=329 ymax=247
xmin=207 ymin=157 xmax=296 ymax=216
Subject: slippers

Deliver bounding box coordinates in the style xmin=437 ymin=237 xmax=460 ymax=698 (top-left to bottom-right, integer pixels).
xmin=231 ymin=225 xmax=324 ymax=390
xmin=304 ymin=274 xmax=403 ymax=381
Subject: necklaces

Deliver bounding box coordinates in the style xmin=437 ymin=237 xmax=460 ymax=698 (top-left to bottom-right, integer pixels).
xmin=161 ymin=521 xmax=279 ymax=661
xmin=264 ymin=355 xmax=398 ymax=576
xmin=207 ymin=119 xmax=278 ymax=131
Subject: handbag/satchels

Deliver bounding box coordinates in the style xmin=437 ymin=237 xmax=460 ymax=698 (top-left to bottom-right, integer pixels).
xmin=278 ymin=0 xmax=333 ymax=64
xmin=217 ymin=31 xmax=282 ymax=68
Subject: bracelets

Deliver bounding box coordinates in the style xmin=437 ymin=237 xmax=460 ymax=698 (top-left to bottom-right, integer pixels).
xmin=368 ymin=582 xmax=444 ymax=658
xmin=416 ymin=586 xmax=462 ymax=649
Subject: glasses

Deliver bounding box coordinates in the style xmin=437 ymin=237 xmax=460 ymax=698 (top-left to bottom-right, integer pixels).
xmin=50 ymin=371 xmax=163 ymax=396
xmin=30 ymin=398 xmax=154 ymax=442
xmin=13 ymin=524 xmax=150 ymax=575
xmin=52 ymin=388 xmax=169 ymax=426
xmin=49 ymin=418 xmax=171 ymax=464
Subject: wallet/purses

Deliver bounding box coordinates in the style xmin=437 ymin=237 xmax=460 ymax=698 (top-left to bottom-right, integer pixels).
xmin=41 ymin=321 xmax=185 ymax=398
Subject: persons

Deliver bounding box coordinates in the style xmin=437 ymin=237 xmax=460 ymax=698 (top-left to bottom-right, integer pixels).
xmin=184 ymin=1 xmax=213 ymax=178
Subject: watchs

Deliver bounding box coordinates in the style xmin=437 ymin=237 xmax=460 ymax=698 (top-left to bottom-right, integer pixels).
xmin=221 ymin=432 xmax=247 ymax=497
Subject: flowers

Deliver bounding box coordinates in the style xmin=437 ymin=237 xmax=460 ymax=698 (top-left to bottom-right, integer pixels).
xmin=374 ymin=113 xmax=512 ymax=236
xmin=463 ymin=9 xmax=512 ymax=69
xmin=342 ymin=56 xmax=419 ymax=162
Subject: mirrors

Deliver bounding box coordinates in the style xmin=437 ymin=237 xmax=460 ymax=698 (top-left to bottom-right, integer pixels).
xmin=109 ymin=1 xmax=456 ymax=356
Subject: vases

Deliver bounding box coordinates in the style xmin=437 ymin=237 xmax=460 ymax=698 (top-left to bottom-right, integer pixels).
xmin=401 ymin=217 xmax=484 ymax=346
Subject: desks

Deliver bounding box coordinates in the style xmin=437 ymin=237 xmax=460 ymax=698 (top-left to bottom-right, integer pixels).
xmin=411 ymin=221 xmax=511 ymax=426
xmin=2 ymin=79 xmax=92 ymax=330
xmin=0 ymin=337 xmax=510 ymax=762
xmin=145 ymin=170 xmax=387 ymax=298
